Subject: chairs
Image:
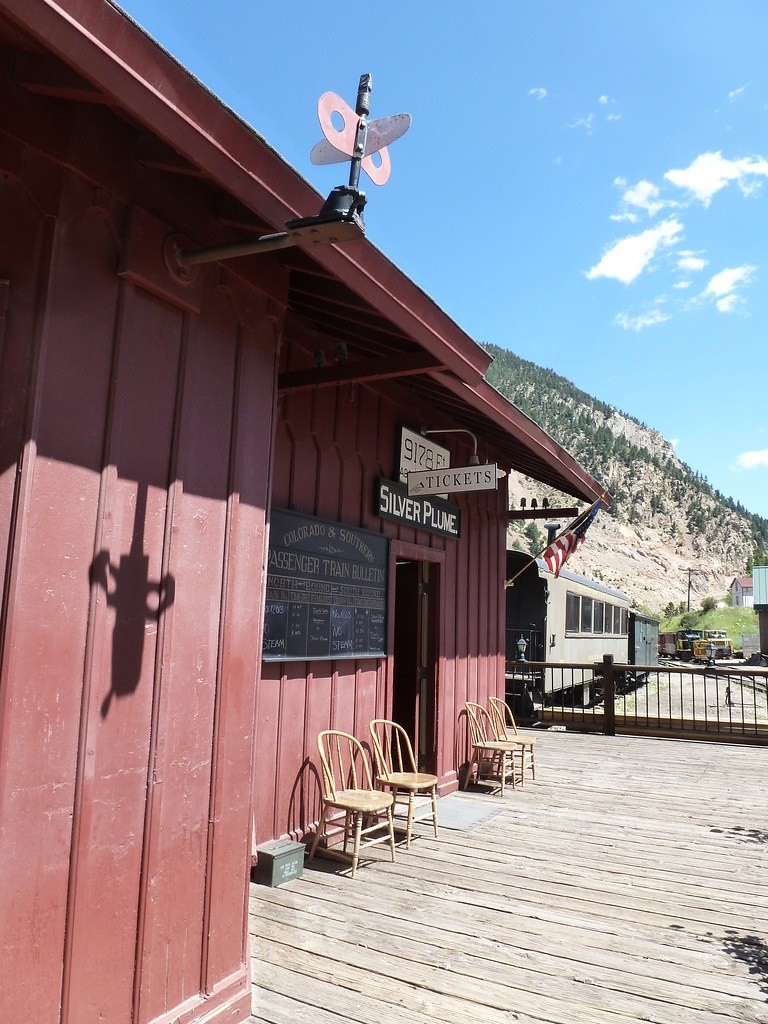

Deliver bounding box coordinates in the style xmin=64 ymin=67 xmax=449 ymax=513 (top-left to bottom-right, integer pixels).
xmin=463 ymin=702 xmax=517 ymax=797
xmin=365 ymin=719 xmax=438 ymax=850
xmin=488 ymin=697 xmax=535 ymax=787
xmin=307 ymin=730 xmax=395 ymax=878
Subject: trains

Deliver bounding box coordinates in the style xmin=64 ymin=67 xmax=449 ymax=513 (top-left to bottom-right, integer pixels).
xmin=505 ymin=524 xmax=734 ymax=709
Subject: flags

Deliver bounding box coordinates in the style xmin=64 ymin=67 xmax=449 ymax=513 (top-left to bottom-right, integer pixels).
xmin=544 ymin=498 xmax=599 ymax=578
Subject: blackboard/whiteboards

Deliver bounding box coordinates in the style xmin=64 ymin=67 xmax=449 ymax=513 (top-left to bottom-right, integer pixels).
xmin=261 ymin=502 xmax=392 ymax=666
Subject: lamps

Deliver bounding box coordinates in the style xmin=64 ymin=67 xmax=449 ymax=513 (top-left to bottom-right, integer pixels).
xmin=704 ymin=640 xmax=718 ymax=668
xmin=420 ymin=425 xmax=507 ymax=479
xmin=516 ymin=634 xmax=528 ymax=662
